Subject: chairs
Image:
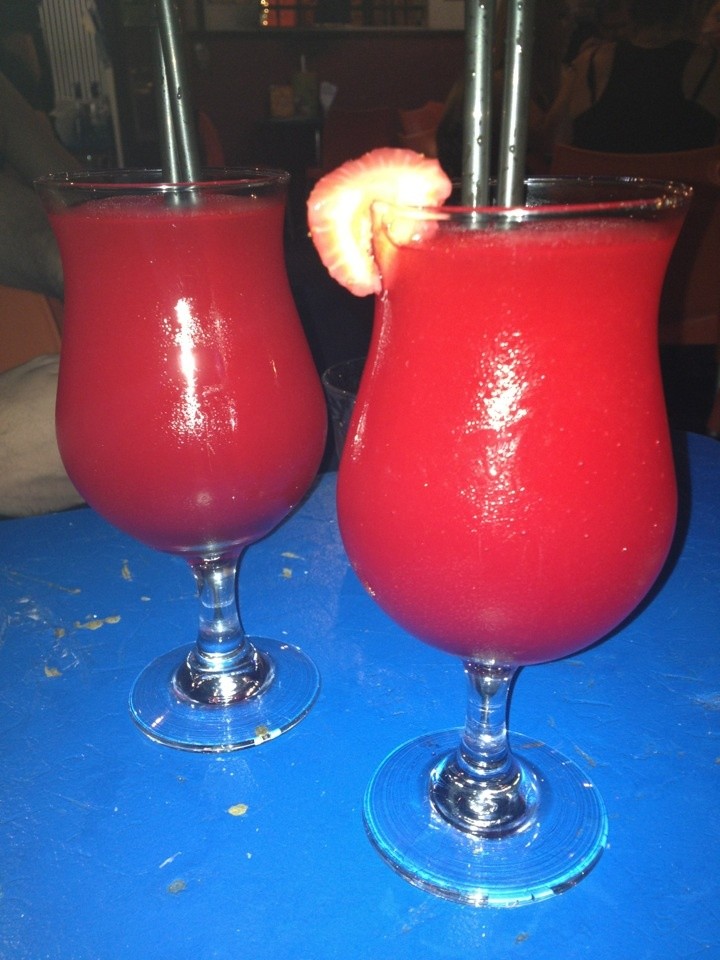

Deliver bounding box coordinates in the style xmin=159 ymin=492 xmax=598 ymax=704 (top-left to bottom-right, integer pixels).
xmin=399 ymin=98 xmax=448 ymax=159
xmin=0 ymin=282 xmax=62 ymax=380
xmin=549 ymin=142 xmax=719 ymax=441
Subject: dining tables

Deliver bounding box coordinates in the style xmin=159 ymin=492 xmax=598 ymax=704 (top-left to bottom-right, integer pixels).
xmin=1 ymin=428 xmax=720 ymax=960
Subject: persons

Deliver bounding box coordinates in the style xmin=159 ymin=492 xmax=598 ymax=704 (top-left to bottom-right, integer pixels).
xmin=1 ymin=74 xmax=87 ymax=300
xmin=541 ymin=1 xmax=719 ymax=440
xmin=571 ymin=3 xmax=631 ymax=64
xmin=438 ymin=1 xmax=578 ymax=207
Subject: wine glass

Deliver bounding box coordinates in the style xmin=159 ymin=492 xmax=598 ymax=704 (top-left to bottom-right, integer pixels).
xmin=334 ymin=176 xmax=690 ymax=910
xmin=38 ymin=153 xmax=330 ymax=753
